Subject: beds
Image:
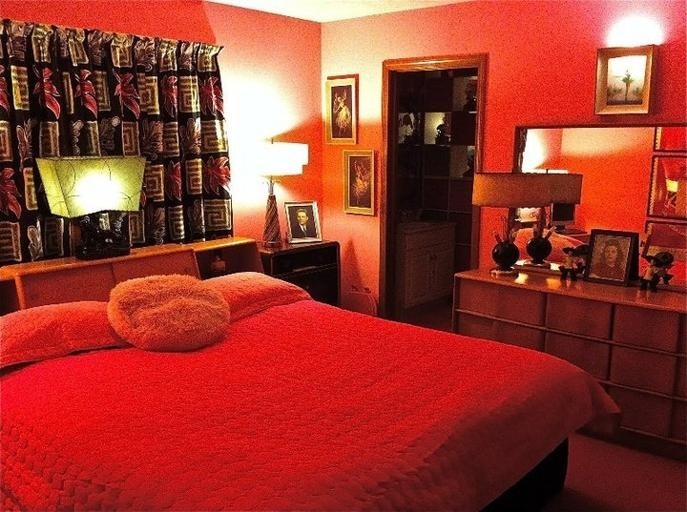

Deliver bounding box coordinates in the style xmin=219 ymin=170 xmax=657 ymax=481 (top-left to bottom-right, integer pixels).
xmin=0 ymin=240 xmax=623 ymax=512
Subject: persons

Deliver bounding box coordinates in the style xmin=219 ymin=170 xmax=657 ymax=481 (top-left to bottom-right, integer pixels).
xmin=290 ymin=209 xmax=316 ymax=238
xmin=352 ymin=160 xmax=368 ymax=206
xmin=333 ymin=89 xmax=352 ymax=137
xmin=593 ymin=239 xmax=626 ymax=278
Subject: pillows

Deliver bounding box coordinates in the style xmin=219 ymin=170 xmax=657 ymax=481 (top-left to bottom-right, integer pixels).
xmin=107 ymin=273 xmax=230 ymax=353
xmin=1 ymin=301 xmax=127 ymax=367
xmin=200 ymin=272 xmax=308 ymax=323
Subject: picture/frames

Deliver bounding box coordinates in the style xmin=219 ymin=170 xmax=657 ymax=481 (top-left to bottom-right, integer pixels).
xmin=646 ymin=152 xmax=687 ymax=225
xmin=594 ymin=44 xmax=656 ymax=116
xmin=324 ymin=78 xmax=357 ymax=145
xmin=343 ymin=149 xmax=375 ymax=216
xmin=584 ymin=228 xmax=638 ymax=287
xmin=653 ymin=126 xmax=687 ymax=153
xmin=283 ymin=200 xmax=322 ymax=244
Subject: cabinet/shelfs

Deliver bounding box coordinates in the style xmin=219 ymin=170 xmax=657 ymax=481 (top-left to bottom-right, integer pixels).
xmin=392 ymin=219 xmax=456 ymax=317
xmin=397 ymin=69 xmax=477 ymax=274
xmin=451 ymin=277 xmax=687 ymax=462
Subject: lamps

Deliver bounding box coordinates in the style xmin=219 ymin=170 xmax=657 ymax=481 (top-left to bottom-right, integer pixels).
xmin=245 ymin=140 xmax=309 ymax=248
xmin=471 ymin=172 xmax=583 ymax=278
xmin=35 ymin=156 xmax=147 ymax=261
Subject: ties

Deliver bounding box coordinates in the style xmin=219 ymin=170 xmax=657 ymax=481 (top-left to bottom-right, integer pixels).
xmin=303 ymin=225 xmax=306 ymax=236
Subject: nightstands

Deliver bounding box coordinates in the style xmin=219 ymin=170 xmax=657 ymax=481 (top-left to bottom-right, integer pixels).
xmin=255 ymin=237 xmax=340 ymax=307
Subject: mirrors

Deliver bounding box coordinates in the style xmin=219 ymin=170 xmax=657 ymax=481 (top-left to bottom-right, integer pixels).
xmin=506 ymin=120 xmax=687 ymax=293
